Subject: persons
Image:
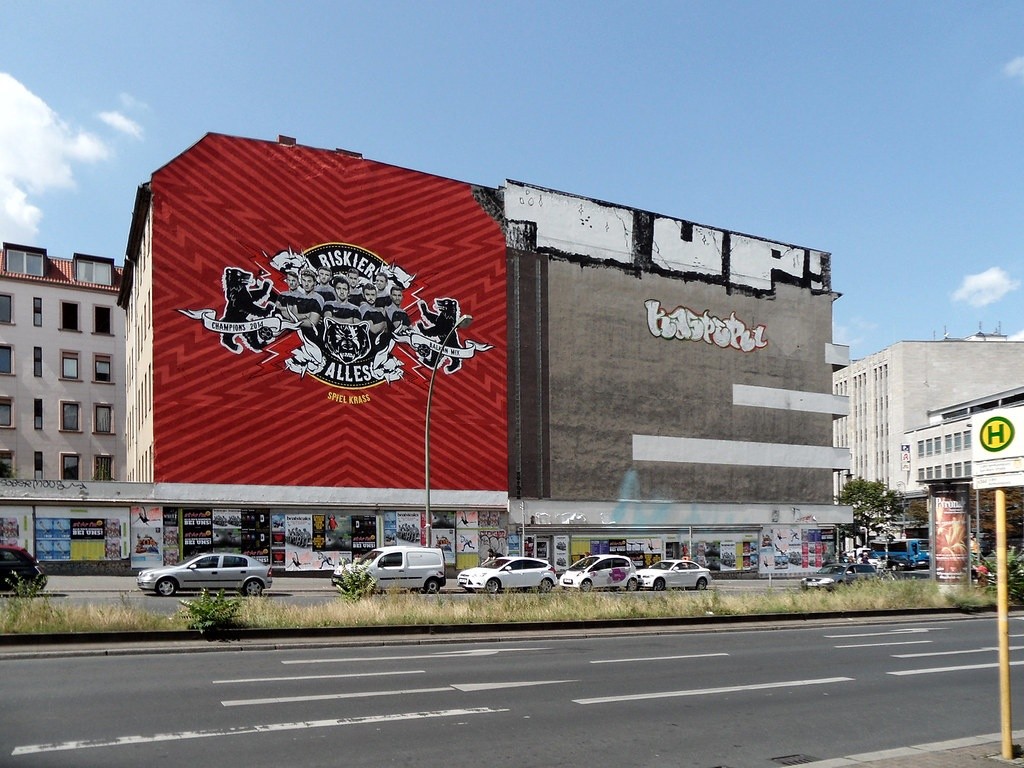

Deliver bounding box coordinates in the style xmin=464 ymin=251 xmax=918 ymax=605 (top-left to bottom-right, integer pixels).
xmin=482 ymin=549 xmax=504 ymax=565
xmin=971 ymin=533 xmax=981 ymax=554
xmin=822 ymin=551 xmax=883 ymax=569
xmin=280 ymin=267 xmax=410 ymax=334
xmin=584 ymin=553 xmax=591 ymax=566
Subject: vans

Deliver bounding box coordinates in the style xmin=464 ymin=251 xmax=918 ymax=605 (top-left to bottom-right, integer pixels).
xmin=331 ymin=545 xmax=446 ymax=594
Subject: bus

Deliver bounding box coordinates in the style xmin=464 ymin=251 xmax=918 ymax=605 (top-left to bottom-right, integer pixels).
xmin=869 ymin=538 xmax=930 ymax=568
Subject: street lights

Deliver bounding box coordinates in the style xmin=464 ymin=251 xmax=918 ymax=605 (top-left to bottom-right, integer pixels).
xmin=896 ymin=481 xmax=906 ymax=538
xmin=837 ymin=470 xmax=855 ymax=562
xmin=425 ymin=315 xmax=475 ymax=547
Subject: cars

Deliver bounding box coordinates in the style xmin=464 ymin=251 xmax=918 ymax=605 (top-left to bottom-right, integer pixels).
xmin=559 ymin=553 xmax=638 ymax=591
xmin=456 ymin=556 xmax=557 ymax=593
xmin=801 ymin=562 xmax=877 ymax=592
xmin=0 ymin=544 xmax=47 ymax=596
xmin=880 ymin=555 xmax=916 ymax=572
xmin=136 ymin=552 xmax=272 ymax=598
xmin=637 ymin=560 xmax=714 ymax=590
